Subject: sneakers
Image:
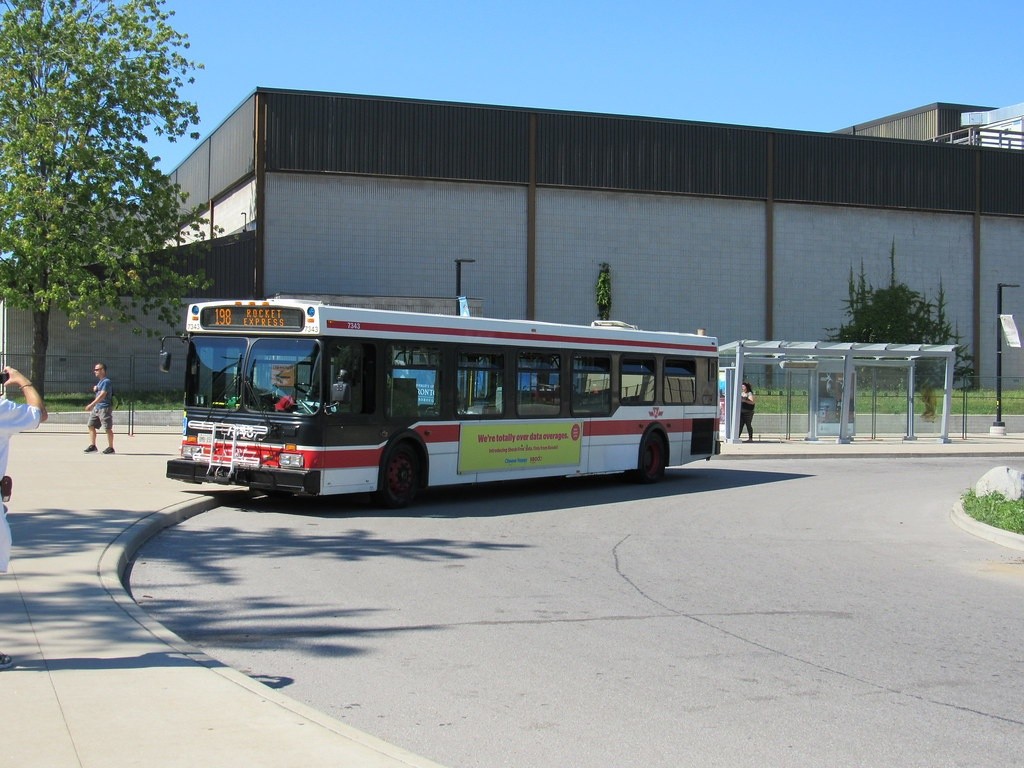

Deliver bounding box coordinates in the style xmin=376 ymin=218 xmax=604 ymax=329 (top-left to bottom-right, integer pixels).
xmin=103 ymin=447 xmax=114 ymax=454
xmin=83 ymin=445 xmax=98 ymax=454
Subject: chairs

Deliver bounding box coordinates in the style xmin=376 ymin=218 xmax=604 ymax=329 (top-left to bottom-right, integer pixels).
xmin=332 ymin=369 xmax=351 ymax=403
xmin=518 ymin=387 xmax=640 ymax=407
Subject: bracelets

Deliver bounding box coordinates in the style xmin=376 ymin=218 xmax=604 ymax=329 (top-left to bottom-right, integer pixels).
xmin=17 ymin=382 xmax=33 ymax=390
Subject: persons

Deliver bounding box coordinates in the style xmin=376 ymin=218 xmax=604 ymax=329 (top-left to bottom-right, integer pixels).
xmin=734 ymin=383 xmax=754 ymax=443
xmin=0 ymin=365 xmax=49 ymax=671
xmin=83 ymin=362 xmax=116 ymax=455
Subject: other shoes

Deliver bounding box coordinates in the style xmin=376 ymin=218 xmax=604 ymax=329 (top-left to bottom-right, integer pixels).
xmin=746 ymin=439 xmax=754 ymax=443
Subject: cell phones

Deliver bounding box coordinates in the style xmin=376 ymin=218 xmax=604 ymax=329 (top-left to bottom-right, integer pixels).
xmin=0 ymin=372 xmax=9 ymax=384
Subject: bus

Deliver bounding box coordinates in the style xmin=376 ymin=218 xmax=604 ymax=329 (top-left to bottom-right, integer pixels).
xmin=157 ymin=299 xmax=726 ymax=521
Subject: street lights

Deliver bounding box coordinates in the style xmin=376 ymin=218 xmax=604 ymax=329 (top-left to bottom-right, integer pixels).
xmin=453 ymin=256 xmax=476 ymax=316
xmin=993 ymin=280 xmax=1022 ymax=426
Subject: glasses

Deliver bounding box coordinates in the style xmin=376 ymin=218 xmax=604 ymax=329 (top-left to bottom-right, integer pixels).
xmin=94 ymin=368 xmax=103 ymax=371
